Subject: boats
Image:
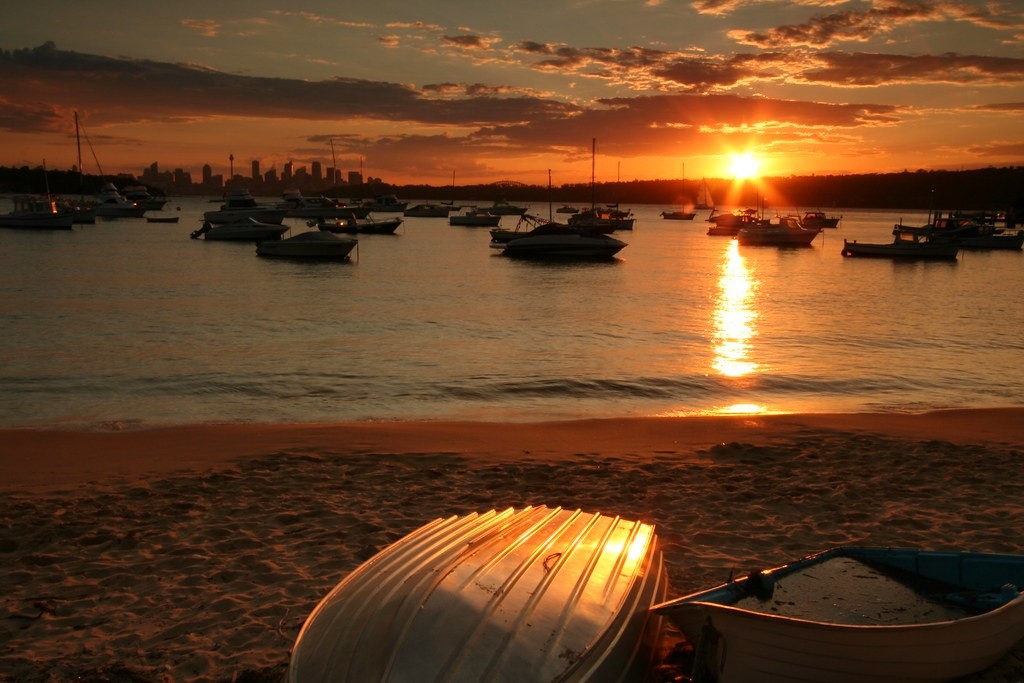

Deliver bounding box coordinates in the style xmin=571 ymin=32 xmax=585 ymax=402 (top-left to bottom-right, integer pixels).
xmin=450 ymin=211 xmax=501 ymax=226
xmin=649 ymin=546 xmax=1024 ymax=683
xmin=126 ymin=191 xmax=167 ymax=209
xmin=189 ymin=189 xmax=461 ymax=261
xmin=842 ymin=206 xmax=1024 ymax=258
xmin=0 ymin=158 xmax=96 ymax=230
xmin=478 ymin=194 xmax=528 ymax=215
xmin=283 ymin=510 xmax=669 ymax=683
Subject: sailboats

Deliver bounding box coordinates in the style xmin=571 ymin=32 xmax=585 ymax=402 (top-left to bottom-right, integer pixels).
xmin=75 ymin=112 xmax=148 ymax=216
xmin=660 ymin=163 xmax=839 ymax=247
xmin=490 ymin=138 xmax=636 ymax=258
